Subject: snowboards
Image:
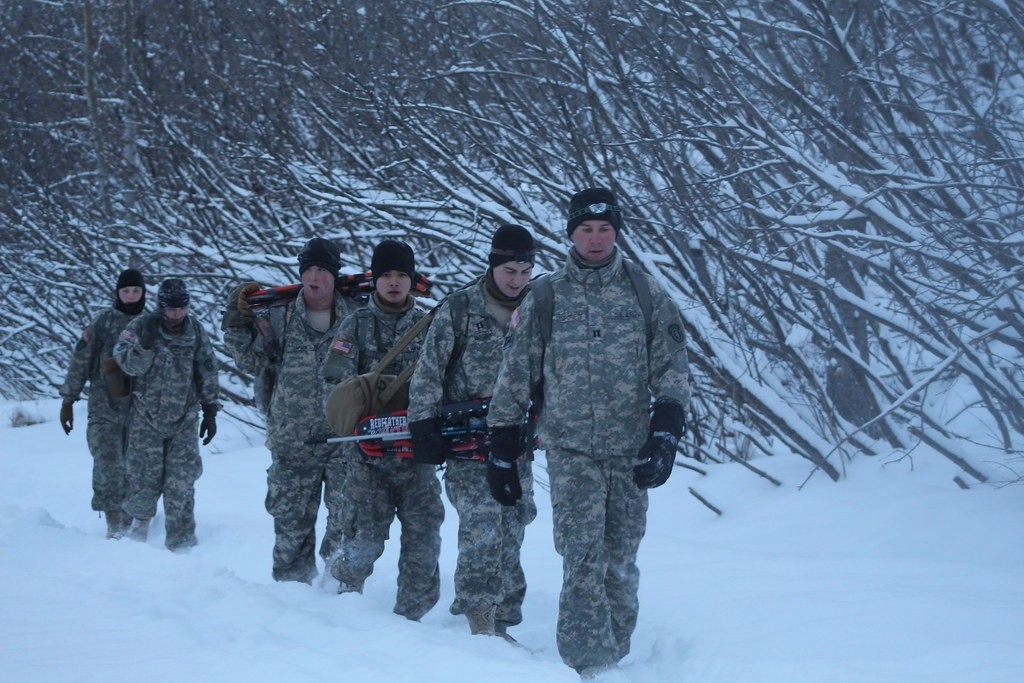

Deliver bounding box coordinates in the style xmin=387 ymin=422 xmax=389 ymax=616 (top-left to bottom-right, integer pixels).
xmin=353 ymin=394 xmax=541 ymax=463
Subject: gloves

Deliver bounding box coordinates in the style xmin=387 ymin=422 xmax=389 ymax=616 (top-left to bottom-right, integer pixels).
xmin=200 ymin=405 xmax=218 ymax=446
xmin=487 ymin=425 xmax=526 ymax=506
xmin=408 ymin=416 xmax=445 ymax=465
xmin=632 ymin=402 xmax=687 ymax=489
xmin=139 ymin=310 xmax=164 ymax=349
xmin=60 ymin=402 xmax=73 ymax=435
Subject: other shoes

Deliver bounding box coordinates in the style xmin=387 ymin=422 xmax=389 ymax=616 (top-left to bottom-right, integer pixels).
xmin=130 ymin=517 xmax=150 ymax=543
xmin=582 ymin=657 xmax=619 ymax=681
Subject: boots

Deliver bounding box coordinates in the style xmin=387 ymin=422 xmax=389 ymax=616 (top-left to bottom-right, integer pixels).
xmin=122 ymin=512 xmax=130 ymax=536
xmin=467 ymin=604 xmax=497 ymax=636
xmin=105 ymin=511 xmax=124 ymax=540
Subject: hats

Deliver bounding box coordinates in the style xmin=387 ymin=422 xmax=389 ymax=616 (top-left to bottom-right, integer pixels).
xmin=489 ymin=224 xmax=534 ymax=269
xmin=157 ymin=279 xmax=189 ymax=310
xmin=567 ymin=187 xmax=620 ymax=241
xmin=116 ymin=269 xmax=146 ymax=314
xmin=371 ymin=240 xmax=415 ymax=290
xmin=298 ymin=238 xmax=341 ymax=278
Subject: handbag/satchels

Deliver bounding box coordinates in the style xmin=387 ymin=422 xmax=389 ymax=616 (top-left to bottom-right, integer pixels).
xmin=328 ymin=291 xmax=460 ymax=437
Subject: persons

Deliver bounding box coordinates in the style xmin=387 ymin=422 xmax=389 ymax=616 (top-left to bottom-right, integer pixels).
xmin=486 ymin=189 xmax=689 ymax=681
xmin=60 ymin=268 xmax=218 ymax=553
xmin=408 ymin=226 xmax=547 ymax=647
xmin=229 ymin=238 xmax=366 ymax=585
xmin=323 ymin=240 xmax=444 ymax=623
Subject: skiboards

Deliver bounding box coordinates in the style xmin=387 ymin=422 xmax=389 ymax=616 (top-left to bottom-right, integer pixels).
xmin=246 ymin=270 xmax=434 ymax=307
